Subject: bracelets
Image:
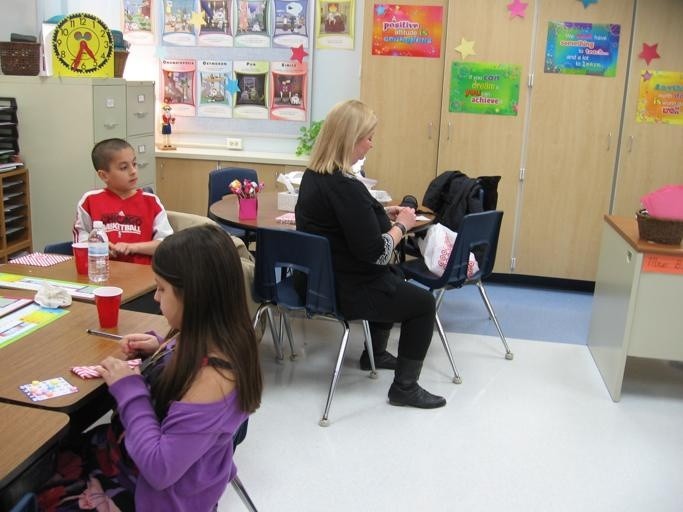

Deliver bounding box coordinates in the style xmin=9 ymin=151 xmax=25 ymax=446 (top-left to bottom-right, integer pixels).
xmin=393 ymin=222 xmax=407 ymax=236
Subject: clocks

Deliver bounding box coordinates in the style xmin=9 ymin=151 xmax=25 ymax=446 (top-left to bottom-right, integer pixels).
xmin=51 ymin=13 xmax=115 ymax=78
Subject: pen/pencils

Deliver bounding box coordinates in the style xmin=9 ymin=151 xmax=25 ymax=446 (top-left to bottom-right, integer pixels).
xmin=86 ymin=328 xmax=123 ymax=340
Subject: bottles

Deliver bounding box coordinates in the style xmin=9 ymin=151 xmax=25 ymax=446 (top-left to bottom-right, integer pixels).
xmin=88 ymin=221 xmax=111 ymax=283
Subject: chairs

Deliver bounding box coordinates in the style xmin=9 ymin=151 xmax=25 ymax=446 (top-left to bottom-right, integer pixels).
xmin=45 ymin=243 xmax=75 ymax=258
xmin=255 ymin=232 xmax=377 ymax=426
xmin=207 ymin=168 xmax=259 ymax=261
xmin=218 ymin=416 xmax=257 ymax=511
xmin=399 ymin=210 xmax=513 ymax=386
xmin=396 ymin=174 xmax=500 ymax=263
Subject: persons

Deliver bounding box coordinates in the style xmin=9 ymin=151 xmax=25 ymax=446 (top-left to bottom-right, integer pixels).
xmin=72 ymin=138 xmax=174 ymax=265
xmin=34 ymin=223 xmax=262 ymax=512
xmin=295 ymin=99 xmax=447 ymax=409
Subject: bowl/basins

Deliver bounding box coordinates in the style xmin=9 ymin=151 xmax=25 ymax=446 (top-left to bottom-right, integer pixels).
xmin=636 ymin=208 xmax=683 ymax=244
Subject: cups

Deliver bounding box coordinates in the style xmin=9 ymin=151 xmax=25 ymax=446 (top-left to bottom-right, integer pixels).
xmin=72 ymin=242 xmax=88 ymax=275
xmin=92 ymin=286 xmax=124 ymax=328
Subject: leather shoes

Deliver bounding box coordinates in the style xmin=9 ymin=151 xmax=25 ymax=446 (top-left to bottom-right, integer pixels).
xmin=387 ymin=383 xmax=446 ymax=408
xmin=359 ymin=348 xmax=399 ymax=371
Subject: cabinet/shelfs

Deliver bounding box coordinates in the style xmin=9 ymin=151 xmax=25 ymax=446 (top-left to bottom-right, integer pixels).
xmin=155 ymin=147 xmax=307 ymax=220
xmin=512 ymin=1 xmax=682 ymax=283
xmin=1 ymin=75 xmax=157 ymax=227
xmin=359 ymin=1 xmax=538 ymax=277
xmin=0 ymin=98 xmax=33 ymax=264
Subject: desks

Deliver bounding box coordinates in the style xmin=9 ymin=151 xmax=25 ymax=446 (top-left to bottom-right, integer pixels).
xmin=0 ymin=286 xmax=175 ymax=416
xmin=207 ymin=193 xmax=435 ymax=254
xmin=0 ymin=401 xmax=71 ymax=496
xmin=585 ymin=216 xmax=683 ymax=403
xmin=0 ymin=253 xmax=159 ymax=307
